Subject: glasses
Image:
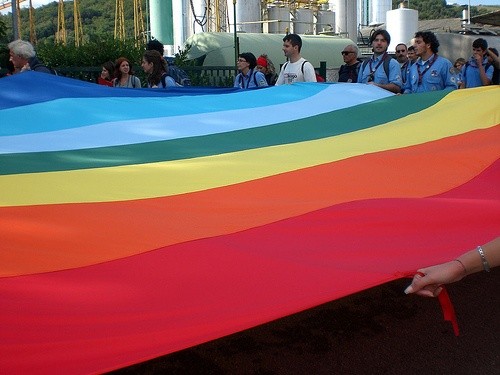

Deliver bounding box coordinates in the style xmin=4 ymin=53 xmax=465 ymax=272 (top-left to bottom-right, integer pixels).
xmin=397 ymin=50 xmax=405 ymax=53
xmin=342 ymin=51 xmax=355 ymax=55
xmin=237 ymin=59 xmax=248 ymax=62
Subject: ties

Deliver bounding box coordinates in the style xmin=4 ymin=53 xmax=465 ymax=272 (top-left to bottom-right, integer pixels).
xmin=370 ymin=53 xmax=386 ymax=73
xmin=417 ymin=54 xmax=437 ymax=87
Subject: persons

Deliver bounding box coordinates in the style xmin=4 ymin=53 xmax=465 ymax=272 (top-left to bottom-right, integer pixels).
xmin=403 ymin=31 xmax=459 ymax=94
xmin=141 ymin=50 xmax=184 ymax=89
xmin=113 ymin=57 xmax=142 ymax=89
xmin=99 ymin=61 xmax=115 ymax=87
xmin=274 ymin=33 xmax=318 ymax=86
xmin=338 ymin=44 xmax=362 ymax=83
xmin=357 ymin=29 xmax=402 ymax=94
xmin=148 ymin=39 xmax=192 ymax=87
xmin=461 ymin=38 xmax=494 ymax=88
xmin=404 ymin=236 xmax=500 ymax=298
xmin=6 ymin=40 xmax=56 ymax=78
xmin=453 ymin=48 xmax=500 ymax=85
xmin=395 ymin=43 xmax=418 ymax=84
xmin=233 ymin=52 xmax=269 ymax=88
xmin=256 ymin=53 xmax=279 ymax=86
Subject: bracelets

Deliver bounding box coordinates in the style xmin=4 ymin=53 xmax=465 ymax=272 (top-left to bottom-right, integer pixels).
xmin=477 ymin=245 xmax=490 ymax=272
xmin=455 ymin=259 xmax=468 ymax=274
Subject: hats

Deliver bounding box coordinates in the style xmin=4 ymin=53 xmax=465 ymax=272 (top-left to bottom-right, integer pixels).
xmin=104 ymin=61 xmax=115 ymax=73
xmin=257 ymin=56 xmax=267 ymax=67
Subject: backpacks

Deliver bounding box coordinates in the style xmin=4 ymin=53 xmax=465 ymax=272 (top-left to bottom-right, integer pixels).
xmin=167 ymin=61 xmax=192 ymax=86
xmin=284 ymin=61 xmax=325 ymax=82
